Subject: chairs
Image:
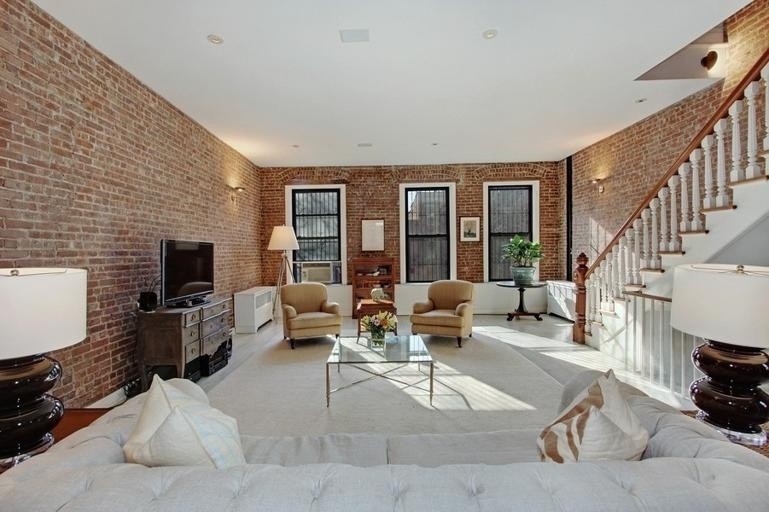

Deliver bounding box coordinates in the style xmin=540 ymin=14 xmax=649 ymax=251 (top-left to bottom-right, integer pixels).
xmin=410 ymin=280 xmax=474 ymax=348
xmin=281 ymin=282 xmax=342 ymax=349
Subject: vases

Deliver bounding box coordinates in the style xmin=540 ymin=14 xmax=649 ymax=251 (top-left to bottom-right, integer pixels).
xmin=371 ymin=334 xmax=386 ymax=348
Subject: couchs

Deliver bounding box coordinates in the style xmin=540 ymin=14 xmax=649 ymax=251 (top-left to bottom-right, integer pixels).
xmin=0 ymin=368 xmax=769 ymax=512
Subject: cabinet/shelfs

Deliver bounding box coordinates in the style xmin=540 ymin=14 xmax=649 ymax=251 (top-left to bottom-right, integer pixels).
xmin=160 ymin=297 xmax=232 ymax=376
xmin=352 ymin=256 xmax=395 ymax=319
xmin=234 ymin=286 xmax=274 ymax=333
xmin=546 ymin=281 xmax=600 ymax=322
xmin=136 ymin=297 xmax=202 ymax=393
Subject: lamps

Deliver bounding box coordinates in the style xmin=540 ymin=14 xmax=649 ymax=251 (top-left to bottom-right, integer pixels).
xmin=231 ymin=182 xmax=245 ymax=201
xmin=267 ymin=223 xmax=300 ymax=320
xmin=701 ymin=51 xmax=717 ymax=69
xmin=592 ymin=178 xmax=604 ymax=193
xmin=670 ymin=263 xmax=769 ymax=446
xmin=1 ymin=267 xmax=87 ymax=468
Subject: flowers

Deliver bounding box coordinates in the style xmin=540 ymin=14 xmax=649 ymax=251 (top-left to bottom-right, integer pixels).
xmin=361 ymin=309 xmax=398 ymax=346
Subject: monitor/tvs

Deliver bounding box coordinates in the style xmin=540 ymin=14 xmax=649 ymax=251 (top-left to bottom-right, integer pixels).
xmin=160 ymin=238 xmax=214 ymax=308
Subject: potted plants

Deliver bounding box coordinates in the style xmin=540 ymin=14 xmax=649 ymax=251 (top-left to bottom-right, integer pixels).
xmin=501 ymin=234 xmax=546 ymax=286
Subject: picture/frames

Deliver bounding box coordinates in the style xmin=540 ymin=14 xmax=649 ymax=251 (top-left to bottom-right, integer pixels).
xmin=361 ymin=218 xmax=385 ymax=252
xmin=459 ymin=216 xmax=481 ymax=242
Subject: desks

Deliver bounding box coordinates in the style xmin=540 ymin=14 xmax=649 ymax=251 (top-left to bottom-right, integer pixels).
xmin=356 ymin=298 xmax=397 ymax=343
xmin=496 ymin=282 xmax=548 ymax=321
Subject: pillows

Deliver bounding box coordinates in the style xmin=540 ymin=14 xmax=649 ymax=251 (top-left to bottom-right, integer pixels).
xmin=123 ymin=373 xmax=248 ymax=470
xmin=534 ymin=369 xmax=651 ymax=463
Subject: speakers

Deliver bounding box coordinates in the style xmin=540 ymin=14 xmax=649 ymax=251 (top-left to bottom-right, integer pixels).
xmin=139 ymin=292 xmax=157 ymax=309
